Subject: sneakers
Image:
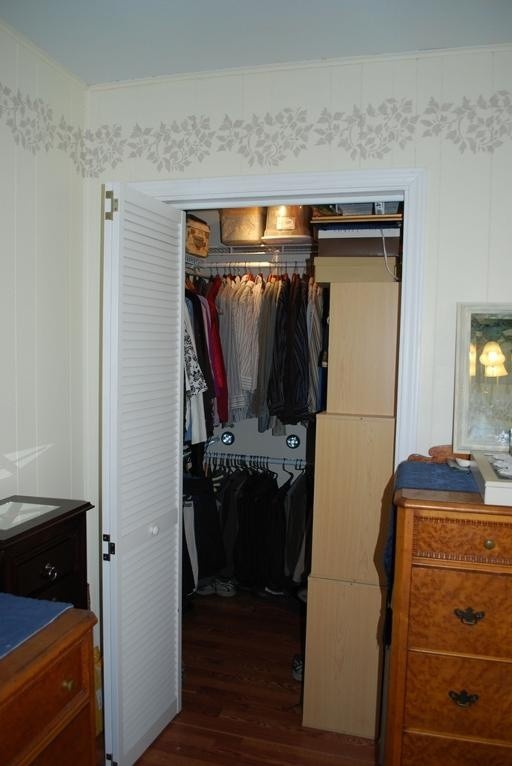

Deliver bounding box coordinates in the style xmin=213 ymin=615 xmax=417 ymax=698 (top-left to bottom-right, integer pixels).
xmin=197 ymin=578 xmax=235 ymax=597
xmin=292 ymin=656 xmax=304 ymax=682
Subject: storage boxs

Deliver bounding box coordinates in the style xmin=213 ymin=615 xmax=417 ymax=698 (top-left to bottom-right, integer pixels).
xmin=469 ymin=450 xmax=511 ymax=509
xmin=218 ymin=207 xmax=265 ymax=245
xmin=316 ymin=229 xmax=398 ymax=256
xmin=182 ymin=215 xmax=212 ymax=258
xmin=262 ymin=205 xmax=311 ymax=244
xmin=312 ymin=256 xmax=397 ymax=283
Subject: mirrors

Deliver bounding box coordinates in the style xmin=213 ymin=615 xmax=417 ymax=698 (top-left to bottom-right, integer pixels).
xmin=450 ymin=301 xmax=511 ymax=455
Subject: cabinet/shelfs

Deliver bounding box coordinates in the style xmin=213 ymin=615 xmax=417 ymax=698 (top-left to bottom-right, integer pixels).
xmin=327 ymin=282 xmax=399 ymax=418
xmin=1 ymin=609 xmax=105 ymax=765
xmin=308 ymin=410 xmax=393 ymax=590
xmin=303 ymin=573 xmax=387 ymax=743
xmin=1 ymin=492 xmax=97 ymax=610
xmin=381 ymin=449 xmax=511 ymax=765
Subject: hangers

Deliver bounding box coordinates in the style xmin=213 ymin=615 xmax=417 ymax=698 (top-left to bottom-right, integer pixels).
xmin=180 ymin=451 xmax=314 ymax=485
xmin=183 ymin=258 xmax=310 ymax=296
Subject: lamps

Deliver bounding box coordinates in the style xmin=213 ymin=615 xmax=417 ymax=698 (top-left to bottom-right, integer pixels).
xmin=469 ymin=315 xmax=509 ymax=385
